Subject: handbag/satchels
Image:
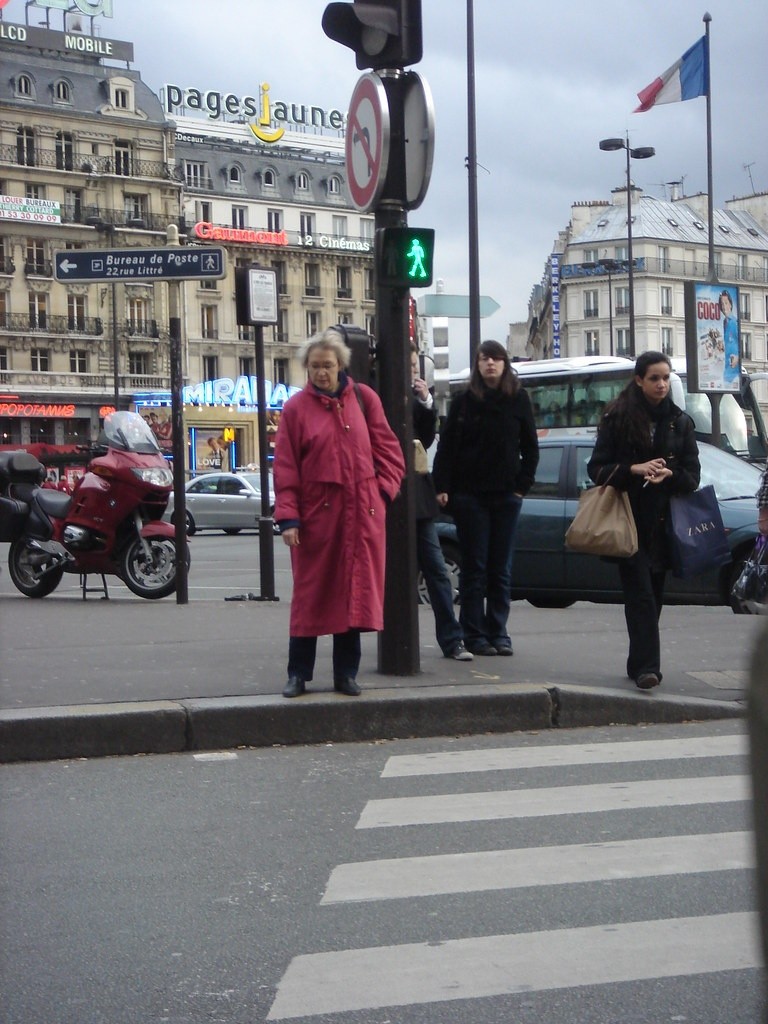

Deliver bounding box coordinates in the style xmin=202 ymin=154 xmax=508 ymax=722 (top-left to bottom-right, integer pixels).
xmin=729 ymin=533 xmax=768 ymax=609
xmin=563 ymin=464 xmax=639 ymax=557
xmin=663 ymin=485 xmax=734 ymax=580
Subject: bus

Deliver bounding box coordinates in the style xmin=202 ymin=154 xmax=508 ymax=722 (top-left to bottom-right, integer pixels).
xmin=447 ymin=355 xmax=768 ymax=472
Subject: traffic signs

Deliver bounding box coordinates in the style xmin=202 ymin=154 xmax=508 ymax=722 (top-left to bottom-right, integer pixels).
xmin=55 ymin=245 xmax=227 ymax=281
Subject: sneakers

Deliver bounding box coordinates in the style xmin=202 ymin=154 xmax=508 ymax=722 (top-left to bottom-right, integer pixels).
xmin=448 ymin=642 xmax=473 ymax=660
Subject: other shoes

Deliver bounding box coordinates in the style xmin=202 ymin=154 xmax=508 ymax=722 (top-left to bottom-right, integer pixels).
xmin=636 ymin=673 xmax=658 ymax=688
xmin=496 ymin=644 xmax=513 ymax=655
xmin=472 ymin=643 xmax=498 ymax=656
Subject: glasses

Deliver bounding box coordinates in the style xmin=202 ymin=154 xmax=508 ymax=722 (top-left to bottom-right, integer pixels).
xmin=306 ymin=361 xmax=339 ymax=371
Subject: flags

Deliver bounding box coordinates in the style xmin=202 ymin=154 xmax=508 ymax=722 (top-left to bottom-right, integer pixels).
xmin=633 ymin=35 xmax=707 ymax=112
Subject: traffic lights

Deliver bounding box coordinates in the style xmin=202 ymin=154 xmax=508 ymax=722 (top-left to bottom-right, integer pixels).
xmin=321 ymin=0 xmax=427 ymax=74
xmin=370 ymin=225 xmax=440 ymax=290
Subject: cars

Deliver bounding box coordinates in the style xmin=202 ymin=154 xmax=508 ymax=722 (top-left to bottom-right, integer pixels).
xmin=159 ymin=470 xmax=282 ymax=537
xmin=412 ymin=431 xmax=768 ymax=613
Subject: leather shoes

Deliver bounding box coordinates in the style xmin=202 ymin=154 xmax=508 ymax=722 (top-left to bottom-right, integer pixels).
xmin=334 ymin=675 xmax=361 ymax=696
xmin=282 ymin=675 xmax=305 ymax=697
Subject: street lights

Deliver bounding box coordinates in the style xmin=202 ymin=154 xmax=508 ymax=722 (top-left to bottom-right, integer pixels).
xmin=583 ymin=257 xmax=635 ymax=356
xmin=597 ymin=129 xmax=658 ymax=358
xmin=87 ymin=215 xmax=146 ymax=408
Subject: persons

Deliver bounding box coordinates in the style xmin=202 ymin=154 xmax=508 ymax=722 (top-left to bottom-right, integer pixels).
xmin=51 ymin=471 xmax=57 ymax=481
xmin=719 ymin=292 xmax=739 ymax=385
xmin=757 ymin=465 xmax=768 ymax=534
xmin=431 ymin=339 xmax=540 ymax=655
xmin=144 ymin=412 xmax=172 ymax=453
xmin=410 ymin=341 xmax=473 ymax=659
xmin=587 ymin=351 xmax=700 ymax=687
xmin=207 ymin=436 xmax=229 ymax=472
xmin=73 ymin=471 xmax=79 ymax=483
xmin=40 ymin=476 xmax=72 ymax=496
xmin=532 ymin=398 xmax=605 ymax=426
xmin=273 ymin=331 xmax=406 ymax=698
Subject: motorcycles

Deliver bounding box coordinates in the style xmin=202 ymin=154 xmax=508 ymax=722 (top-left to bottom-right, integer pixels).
xmin=0 ymin=410 xmax=192 ymax=603
